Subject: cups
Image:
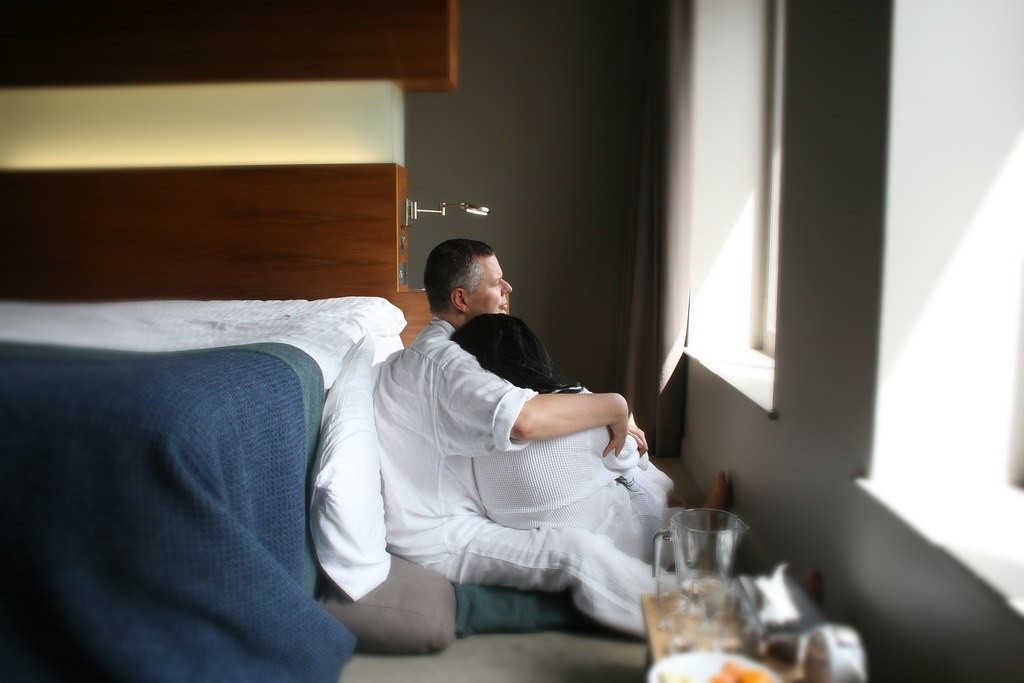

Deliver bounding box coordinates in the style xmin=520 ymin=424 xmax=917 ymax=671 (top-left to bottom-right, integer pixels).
xmin=642 ymin=575 xmax=730 ymax=659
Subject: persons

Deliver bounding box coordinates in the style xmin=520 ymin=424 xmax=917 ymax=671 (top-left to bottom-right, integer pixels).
xmin=450 ymin=312 xmax=674 ymax=566
xmin=373 ymin=238 xmax=821 ymax=639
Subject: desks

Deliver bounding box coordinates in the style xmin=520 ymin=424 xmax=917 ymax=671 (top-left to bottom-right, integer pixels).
xmin=642 ymin=589 xmax=809 ymax=683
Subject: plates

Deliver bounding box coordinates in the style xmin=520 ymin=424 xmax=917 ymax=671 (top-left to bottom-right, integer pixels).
xmin=647 ymin=651 xmax=778 ymax=683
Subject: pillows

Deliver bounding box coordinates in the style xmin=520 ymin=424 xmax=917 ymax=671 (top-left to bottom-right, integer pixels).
xmin=306 ymin=329 xmax=391 ymax=603
xmin=318 ymin=554 xmax=456 ymax=655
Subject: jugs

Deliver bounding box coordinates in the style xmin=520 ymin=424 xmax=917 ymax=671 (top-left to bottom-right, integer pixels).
xmin=654 ymin=508 xmax=750 ymax=618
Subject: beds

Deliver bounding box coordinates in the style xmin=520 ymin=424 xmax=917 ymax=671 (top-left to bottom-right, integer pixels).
xmin=0 ymin=295 xmax=410 ymax=683
